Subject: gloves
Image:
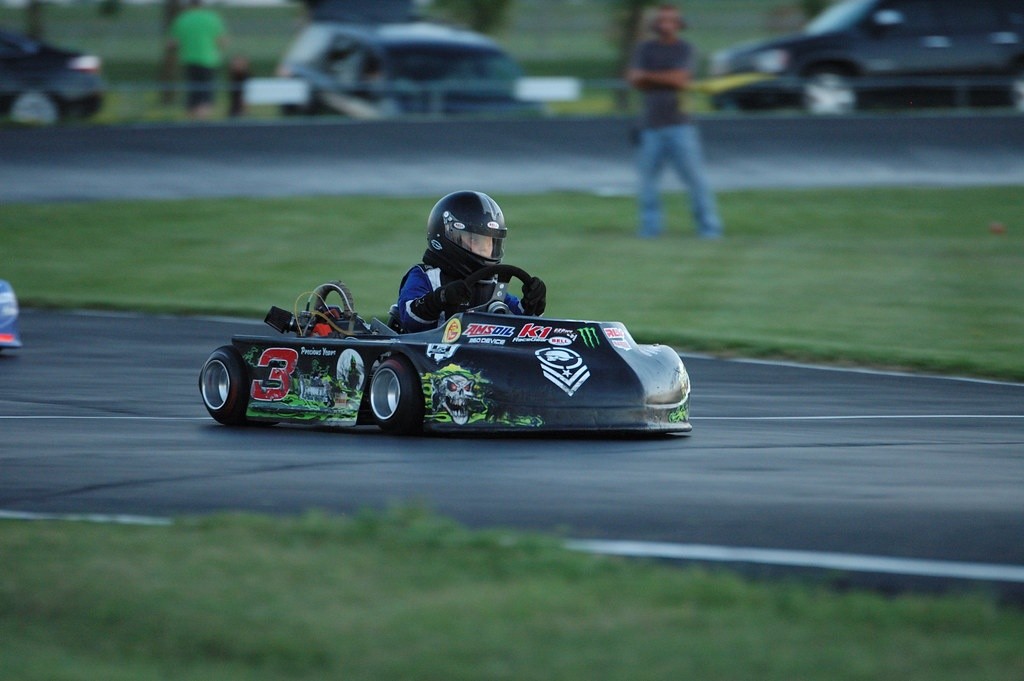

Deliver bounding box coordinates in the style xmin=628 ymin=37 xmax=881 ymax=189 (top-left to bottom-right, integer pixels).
xmin=520 ymin=276 xmax=546 ymax=316
xmin=434 ymin=280 xmax=471 ymax=311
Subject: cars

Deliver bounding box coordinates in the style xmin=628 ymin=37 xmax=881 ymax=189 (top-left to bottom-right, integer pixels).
xmin=275 ymin=22 xmax=542 ymax=118
xmin=707 ymin=0 xmax=1024 ymax=115
xmin=0 ymin=24 xmax=104 ymax=125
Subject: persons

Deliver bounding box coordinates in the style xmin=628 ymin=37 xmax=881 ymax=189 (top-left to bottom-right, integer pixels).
xmin=625 ymin=6 xmax=725 ymax=244
xmin=168 ymin=0 xmax=261 ymax=121
xmin=394 ymin=189 xmax=549 ymax=331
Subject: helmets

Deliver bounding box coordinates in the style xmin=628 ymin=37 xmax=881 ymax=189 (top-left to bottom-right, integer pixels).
xmin=427 ymin=189 xmax=508 ymax=276
